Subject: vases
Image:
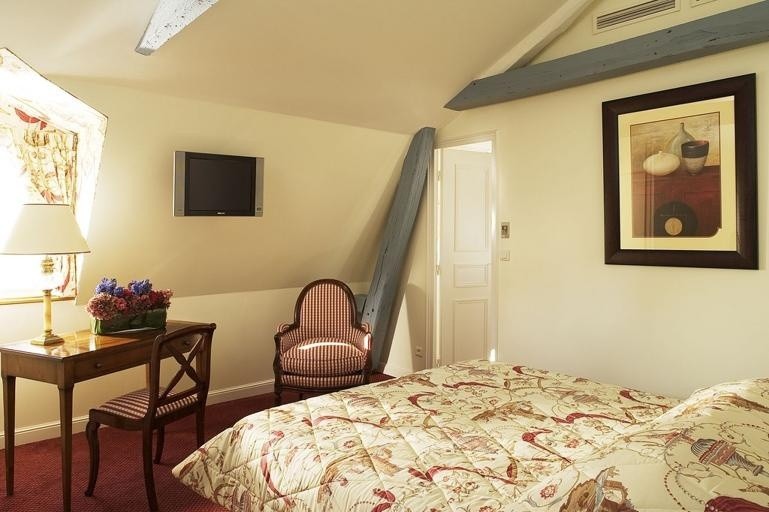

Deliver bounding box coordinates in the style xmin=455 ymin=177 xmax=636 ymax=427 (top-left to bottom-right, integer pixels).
xmin=90 ymin=308 xmax=167 ymax=337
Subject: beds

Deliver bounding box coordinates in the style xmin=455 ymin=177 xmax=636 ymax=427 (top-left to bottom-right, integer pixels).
xmin=176 ymin=361 xmax=769 ymax=512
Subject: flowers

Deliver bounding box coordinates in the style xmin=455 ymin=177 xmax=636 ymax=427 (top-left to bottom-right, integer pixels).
xmin=86 ymin=276 xmax=173 ymax=322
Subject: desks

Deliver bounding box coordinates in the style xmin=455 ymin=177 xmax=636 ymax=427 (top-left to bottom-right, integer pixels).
xmin=1 ymin=319 xmax=211 ymax=511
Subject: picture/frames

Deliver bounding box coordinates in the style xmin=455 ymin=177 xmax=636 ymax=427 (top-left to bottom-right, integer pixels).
xmin=601 ymin=71 xmax=760 ymax=271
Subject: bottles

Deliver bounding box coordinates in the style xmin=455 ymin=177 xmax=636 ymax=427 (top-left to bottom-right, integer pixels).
xmin=669 ymin=122 xmax=696 ymax=157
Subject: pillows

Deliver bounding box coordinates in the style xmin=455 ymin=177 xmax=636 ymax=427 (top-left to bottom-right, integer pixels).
xmin=502 ymin=377 xmax=768 ymax=512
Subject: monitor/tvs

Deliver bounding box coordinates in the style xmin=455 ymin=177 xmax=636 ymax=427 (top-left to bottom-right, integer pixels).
xmin=173 ymin=149 xmax=265 ymax=217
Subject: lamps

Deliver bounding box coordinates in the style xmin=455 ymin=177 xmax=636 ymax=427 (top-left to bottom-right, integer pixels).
xmin=2 ymin=201 xmax=91 ymax=347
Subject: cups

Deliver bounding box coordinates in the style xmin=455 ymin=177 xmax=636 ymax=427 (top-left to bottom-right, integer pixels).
xmin=680 ymin=139 xmax=711 ymax=176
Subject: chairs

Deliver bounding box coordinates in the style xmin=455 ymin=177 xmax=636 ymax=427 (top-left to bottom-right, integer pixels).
xmin=270 ymin=279 xmax=373 ymax=406
xmin=85 ymin=322 xmax=218 ymax=510
